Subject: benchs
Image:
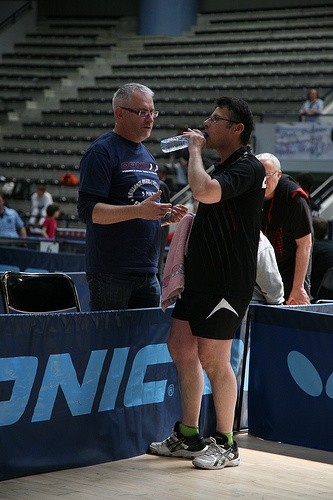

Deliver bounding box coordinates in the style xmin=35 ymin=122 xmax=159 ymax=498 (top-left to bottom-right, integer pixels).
xmin=0 ymin=10 xmax=333 ymax=239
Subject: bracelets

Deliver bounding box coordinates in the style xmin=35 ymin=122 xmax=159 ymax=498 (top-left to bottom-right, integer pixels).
xmin=163 ymin=212 xmax=170 ymax=226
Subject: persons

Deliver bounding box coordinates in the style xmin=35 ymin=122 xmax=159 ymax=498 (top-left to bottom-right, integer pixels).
xmin=149 ymin=97 xmax=267 ymax=469
xmin=77 ymin=82 xmax=188 ymax=312
xmin=0 ymin=168 xmax=80 ymax=242
xmin=299 ymin=90 xmax=324 ymax=125
xmin=157 ymin=130 xmax=328 ymax=305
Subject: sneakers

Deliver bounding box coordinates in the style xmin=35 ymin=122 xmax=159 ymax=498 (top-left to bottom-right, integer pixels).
xmin=191 ymin=432 xmax=242 ymax=470
xmin=148 ymin=420 xmax=207 ymax=457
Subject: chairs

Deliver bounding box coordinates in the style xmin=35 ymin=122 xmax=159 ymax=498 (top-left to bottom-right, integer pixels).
xmin=1 ymin=271 xmax=81 ymax=314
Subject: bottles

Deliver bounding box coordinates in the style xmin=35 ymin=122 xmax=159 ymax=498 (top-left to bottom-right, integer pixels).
xmin=160 ymin=132 xmax=207 ymax=153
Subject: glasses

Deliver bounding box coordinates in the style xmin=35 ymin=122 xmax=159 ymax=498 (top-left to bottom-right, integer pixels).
xmin=206 ymin=114 xmax=243 ymax=125
xmin=265 ymin=170 xmax=278 ymax=179
xmin=120 ymin=106 xmax=159 ymax=119
